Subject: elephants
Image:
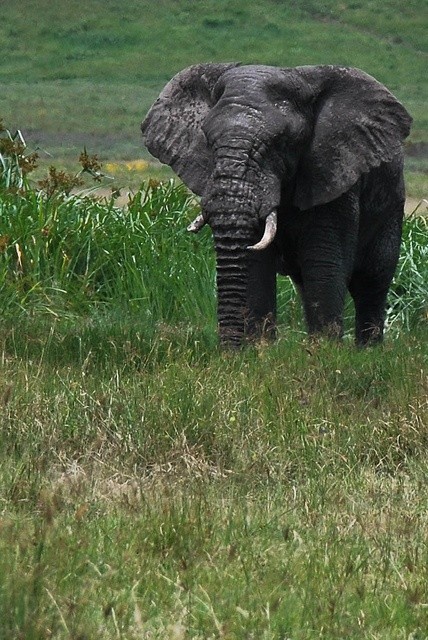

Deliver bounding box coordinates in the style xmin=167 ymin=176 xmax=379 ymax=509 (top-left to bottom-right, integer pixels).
xmin=139 ymin=61 xmax=412 ymax=355
